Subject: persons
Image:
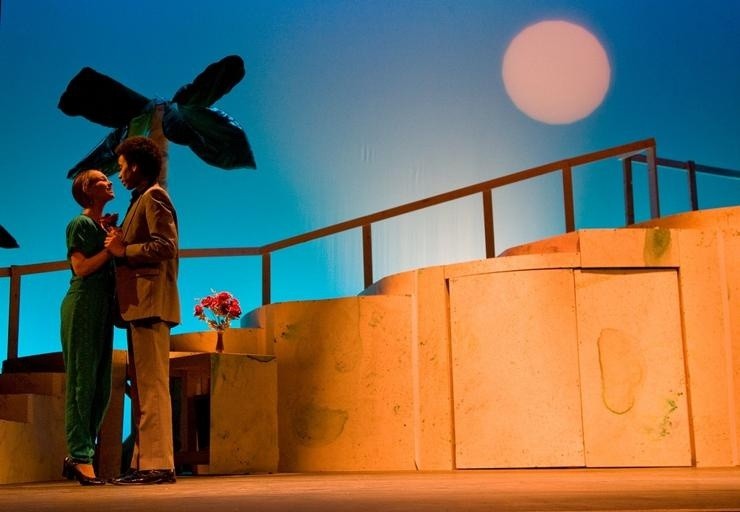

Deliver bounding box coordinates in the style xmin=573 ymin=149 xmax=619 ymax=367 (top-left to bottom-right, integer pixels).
xmin=102 ymin=135 xmax=181 ymax=487
xmin=58 ymin=168 xmax=121 ymax=487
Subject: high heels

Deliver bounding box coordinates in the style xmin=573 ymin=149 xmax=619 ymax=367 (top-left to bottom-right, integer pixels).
xmin=61 ymin=456 xmax=105 ymax=486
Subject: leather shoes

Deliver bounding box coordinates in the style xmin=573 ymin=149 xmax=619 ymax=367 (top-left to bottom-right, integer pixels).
xmin=113 ymin=468 xmax=177 ymax=486
xmin=107 ymin=470 xmax=136 ymax=484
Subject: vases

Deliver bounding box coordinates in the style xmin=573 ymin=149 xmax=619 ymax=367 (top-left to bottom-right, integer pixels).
xmin=216 ymin=329 xmax=225 ymax=352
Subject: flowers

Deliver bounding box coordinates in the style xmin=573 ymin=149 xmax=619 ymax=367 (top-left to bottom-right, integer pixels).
xmin=194 ymin=284 xmax=244 ymax=331
